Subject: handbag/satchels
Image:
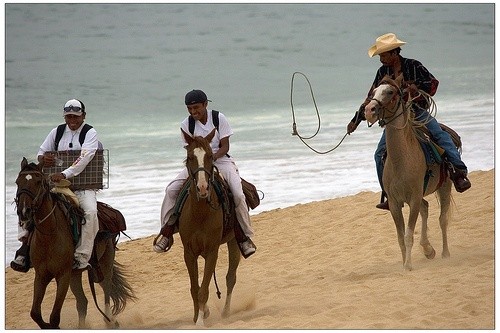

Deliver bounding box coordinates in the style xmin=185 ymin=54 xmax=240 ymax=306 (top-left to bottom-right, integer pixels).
xmin=241 ymin=178 xmax=260 ymax=210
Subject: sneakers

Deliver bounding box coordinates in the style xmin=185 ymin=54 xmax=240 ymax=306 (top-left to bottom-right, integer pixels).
xmin=10 ymin=256 xmax=26 ymax=271
xmin=72 ymin=253 xmax=92 ymax=271
xmin=154 ymin=237 xmax=170 ymax=252
xmin=240 ymin=240 xmax=255 ymax=255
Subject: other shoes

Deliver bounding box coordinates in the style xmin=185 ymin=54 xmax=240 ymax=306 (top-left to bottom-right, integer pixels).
xmin=376 ymin=198 xmax=404 ymax=209
xmin=451 ymin=172 xmax=471 ymax=193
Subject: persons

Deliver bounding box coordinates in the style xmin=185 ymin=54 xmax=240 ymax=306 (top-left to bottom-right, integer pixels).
xmin=347 ymin=33 xmax=471 ymax=210
xmin=10 ymin=98 xmax=103 ymax=270
xmin=153 ymin=90 xmax=256 ymax=256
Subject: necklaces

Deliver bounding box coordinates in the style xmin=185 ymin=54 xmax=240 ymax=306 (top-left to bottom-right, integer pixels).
xmin=69 ymin=130 xmax=76 ymax=148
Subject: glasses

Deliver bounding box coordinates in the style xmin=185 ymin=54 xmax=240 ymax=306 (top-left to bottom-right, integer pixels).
xmin=63 ymin=107 xmax=83 ymax=113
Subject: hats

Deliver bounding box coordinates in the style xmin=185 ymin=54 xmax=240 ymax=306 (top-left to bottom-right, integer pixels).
xmin=63 ymin=99 xmax=85 ymax=116
xmin=185 ymin=89 xmax=212 ymax=105
xmin=369 ymin=33 xmax=406 ymax=57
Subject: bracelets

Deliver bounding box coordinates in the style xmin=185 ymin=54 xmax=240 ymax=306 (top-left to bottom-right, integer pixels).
xmin=61 ymin=173 xmax=66 ymax=179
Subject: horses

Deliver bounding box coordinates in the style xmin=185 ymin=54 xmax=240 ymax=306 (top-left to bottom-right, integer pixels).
xmin=14 ymin=156 xmax=140 ymax=329
xmin=177 ymin=126 xmax=242 ymax=330
xmin=365 ymin=71 xmax=457 ymax=271
